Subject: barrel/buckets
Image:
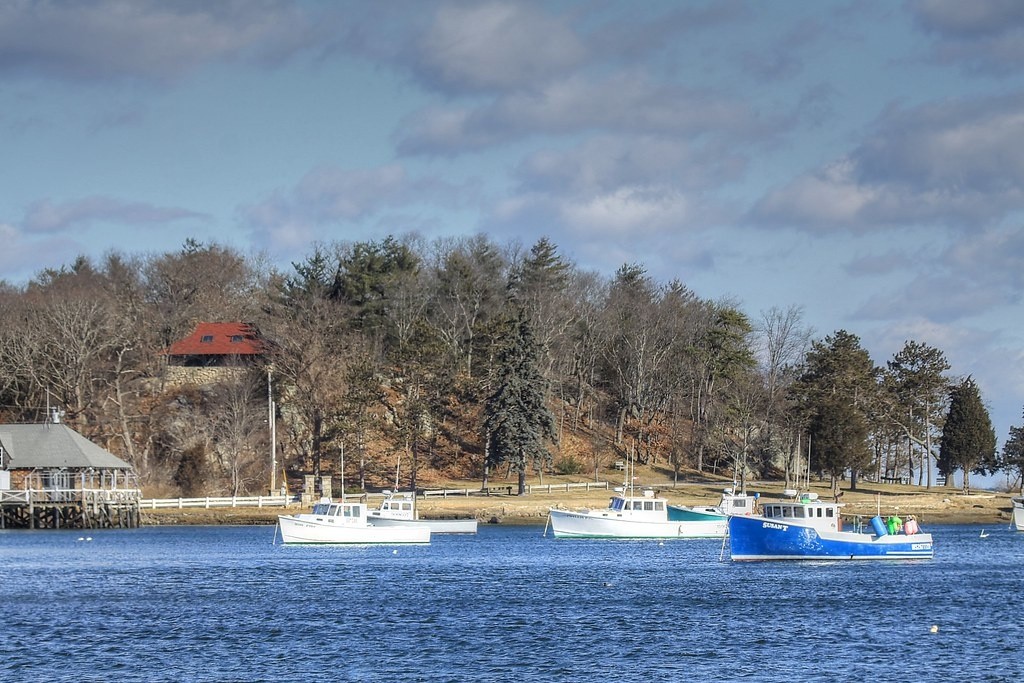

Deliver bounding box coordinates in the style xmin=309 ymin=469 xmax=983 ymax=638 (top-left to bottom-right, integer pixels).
xmin=871 ymin=515 xmax=887 ymax=538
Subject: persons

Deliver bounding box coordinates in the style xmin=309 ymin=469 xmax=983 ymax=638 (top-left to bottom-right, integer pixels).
xmin=280 ymin=481 xmax=286 ymax=507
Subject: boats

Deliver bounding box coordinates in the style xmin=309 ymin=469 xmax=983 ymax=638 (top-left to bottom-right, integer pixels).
xmin=727 ymin=432 xmax=934 ymax=560
xmin=549 ymin=439 xmax=733 ymax=541
xmin=1012 ymin=486 xmax=1024 ymax=533
xmin=278 ymin=496 xmax=478 ymax=544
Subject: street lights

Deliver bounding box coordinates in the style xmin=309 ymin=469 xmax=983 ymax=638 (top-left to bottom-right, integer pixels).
xmin=262 ymin=364 xmax=275 ymax=458
